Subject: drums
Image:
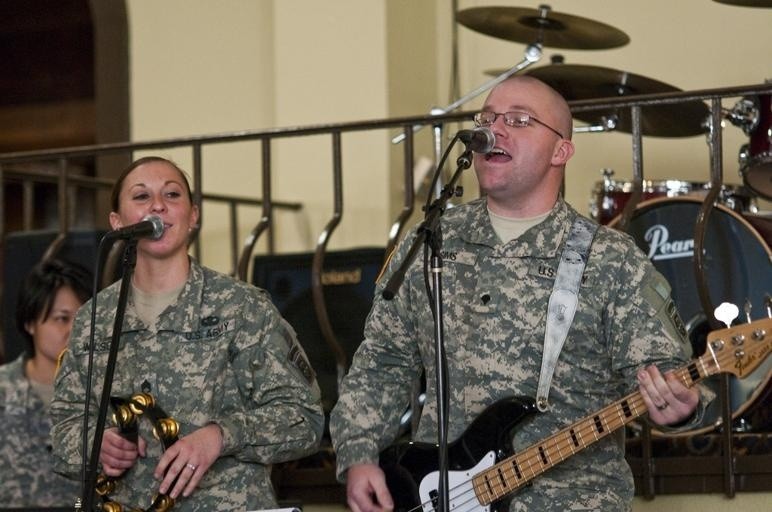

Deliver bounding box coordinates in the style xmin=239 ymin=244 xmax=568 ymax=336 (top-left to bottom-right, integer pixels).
xmin=590 ymin=169 xmax=751 ymax=228
xmin=730 ymin=80 xmax=772 ymax=199
xmin=607 ymin=194 xmax=772 ymax=438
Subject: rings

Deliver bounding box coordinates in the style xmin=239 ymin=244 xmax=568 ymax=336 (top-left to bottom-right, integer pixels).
xmin=656 ymin=402 xmax=670 ymax=412
xmin=184 ymin=464 xmax=199 ymax=472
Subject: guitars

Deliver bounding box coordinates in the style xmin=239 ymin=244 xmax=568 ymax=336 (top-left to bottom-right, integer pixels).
xmin=370 ymin=293 xmax=772 ymax=512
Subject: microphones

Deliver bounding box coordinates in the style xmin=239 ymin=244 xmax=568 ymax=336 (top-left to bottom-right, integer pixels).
xmin=458 ymin=127 xmax=495 ymax=154
xmin=108 ymin=215 xmax=164 ymax=241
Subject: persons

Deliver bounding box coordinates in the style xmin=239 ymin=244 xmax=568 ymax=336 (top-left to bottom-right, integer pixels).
xmin=0 ymin=256 xmax=103 ymax=512
xmin=44 ymin=155 xmax=327 ymax=511
xmin=324 ymin=71 xmax=719 ymax=512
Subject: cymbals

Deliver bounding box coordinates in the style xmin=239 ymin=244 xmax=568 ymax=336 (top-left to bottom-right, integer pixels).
xmin=524 ymin=63 xmax=711 ymax=136
xmin=454 ymin=7 xmax=630 ymax=48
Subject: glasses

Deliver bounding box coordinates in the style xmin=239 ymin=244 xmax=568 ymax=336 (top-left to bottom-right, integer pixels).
xmin=474 ymin=112 xmax=566 ymax=139
